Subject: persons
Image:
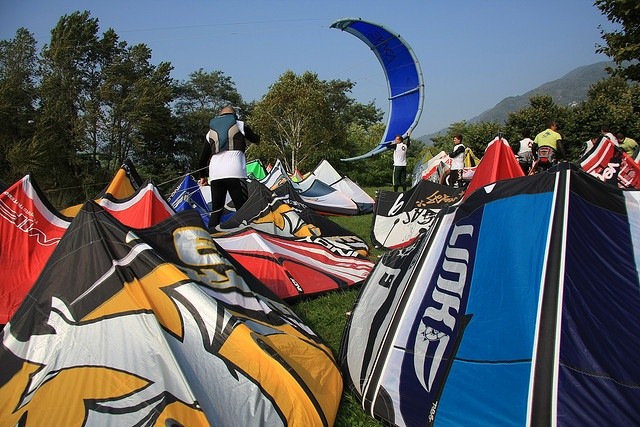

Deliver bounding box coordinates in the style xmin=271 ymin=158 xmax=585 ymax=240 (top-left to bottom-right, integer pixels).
xmin=198 ymin=106 xmax=260 ymax=228
xmin=532 ymin=121 xmax=567 ymax=167
xmin=386 ymin=132 xmax=412 ymax=192
xmin=599 ymin=126 xmax=618 ymax=145
xmin=516 ymin=129 xmax=534 ymax=169
xmin=579 ymin=137 xmax=598 ymax=160
xmin=449 ymin=135 xmax=466 ymax=187
xmin=616 ymin=133 xmax=639 ymax=162
